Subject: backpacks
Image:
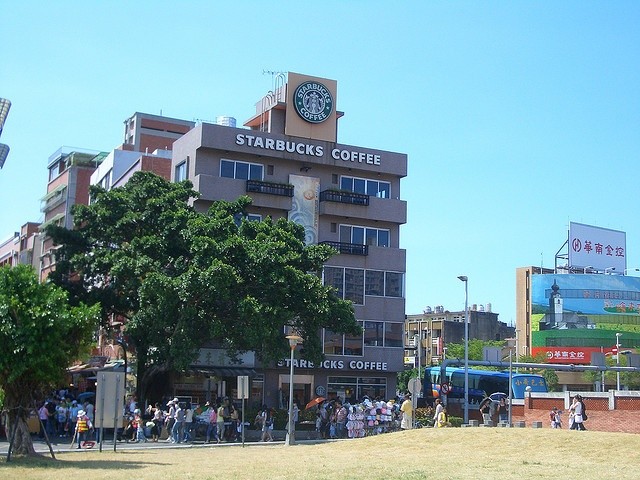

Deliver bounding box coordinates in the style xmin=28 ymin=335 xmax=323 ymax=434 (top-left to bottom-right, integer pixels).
xmin=549 ymin=414 xmax=552 ymax=420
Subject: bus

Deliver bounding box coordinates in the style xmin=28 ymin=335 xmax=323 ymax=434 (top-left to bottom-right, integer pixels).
xmin=423 ymin=367 xmax=548 ymax=405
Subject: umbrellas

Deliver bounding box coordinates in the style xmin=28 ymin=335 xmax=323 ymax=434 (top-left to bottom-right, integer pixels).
xmin=479 ymin=397 xmax=492 ymax=408
xmin=305 ymin=397 xmax=326 ymax=411
xmin=489 ymin=392 xmax=507 ymax=400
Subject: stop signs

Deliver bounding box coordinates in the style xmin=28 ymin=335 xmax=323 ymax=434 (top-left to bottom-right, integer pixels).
xmin=612 ymin=345 xmax=618 ymax=355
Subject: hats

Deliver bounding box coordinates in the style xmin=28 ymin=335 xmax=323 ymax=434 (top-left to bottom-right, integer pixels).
xmin=205 ymin=401 xmax=210 ymax=406
xmin=61 ymin=397 xmax=66 ymax=400
xmin=44 ymin=402 xmax=48 ymax=407
xmin=346 ymin=407 xmax=365 ymax=437
xmin=72 ymin=400 xmax=77 ymax=404
xmin=77 ymin=410 xmax=86 ymax=417
xmin=172 ymin=398 xmax=178 ymax=402
xmin=366 ymin=399 xmax=393 ymax=426
xmin=166 ymin=401 xmax=174 ymax=406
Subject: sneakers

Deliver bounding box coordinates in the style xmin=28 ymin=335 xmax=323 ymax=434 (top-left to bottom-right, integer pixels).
xmin=76 ymin=445 xmax=80 ymax=449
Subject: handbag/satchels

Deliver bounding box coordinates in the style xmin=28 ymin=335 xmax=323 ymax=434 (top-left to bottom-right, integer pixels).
xmin=583 ymin=413 xmax=587 ymax=421
xmin=575 ymin=413 xmax=582 ymax=423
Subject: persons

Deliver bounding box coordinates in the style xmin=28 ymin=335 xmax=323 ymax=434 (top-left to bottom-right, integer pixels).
xmin=549 ymin=406 xmax=557 ymax=428
xmin=438 ymin=407 xmax=451 ymax=427
xmin=285 ymin=401 xmax=299 ymax=431
xmin=255 ymin=404 xmax=275 ymax=442
xmin=316 ymin=401 xmax=347 ymax=439
xmin=497 ymin=398 xmax=509 ymax=420
xmin=570 ymin=394 xmax=588 ymax=430
xmin=480 ymin=400 xmax=491 ymax=421
xmin=38 ymin=389 xmax=94 ymax=449
xmin=424 ymin=404 xmax=435 ymax=426
xmin=568 ymin=403 xmax=580 ymax=430
xmin=120 ymin=398 xmax=241 ymax=444
xmin=555 ymin=410 xmax=562 ymax=429
xmin=579 ymin=395 xmax=587 ymax=421
xmin=433 ymin=398 xmax=443 ymax=427
xmin=400 ymin=394 xmax=413 ymax=430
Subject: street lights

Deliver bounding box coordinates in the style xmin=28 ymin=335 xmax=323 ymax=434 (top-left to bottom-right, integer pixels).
xmin=457 ymin=276 xmax=468 ymax=424
xmin=616 ymin=333 xmax=622 ymax=390
xmin=505 ymin=338 xmax=519 ymax=427
xmin=285 ymin=336 xmax=301 ymax=445
xmin=107 ymin=339 xmax=126 ymax=406
